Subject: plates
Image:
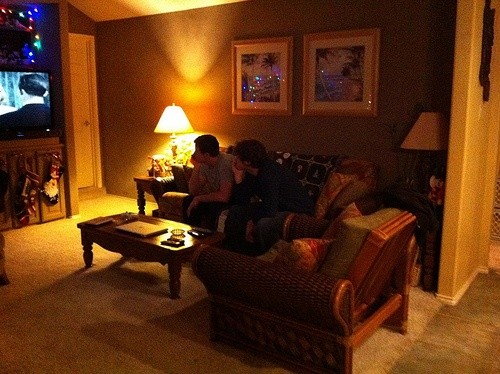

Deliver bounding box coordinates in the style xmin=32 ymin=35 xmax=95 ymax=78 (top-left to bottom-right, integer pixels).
xmin=171 ymin=230 xmax=185 ymax=236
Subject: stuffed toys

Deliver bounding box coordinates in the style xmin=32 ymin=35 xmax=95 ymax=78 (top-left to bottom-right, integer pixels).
xmin=428 ymin=161 xmax=445 ymax=209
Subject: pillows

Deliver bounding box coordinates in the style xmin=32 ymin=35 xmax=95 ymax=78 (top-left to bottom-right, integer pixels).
xmin=170 ymin=164 xmax=194 ymax=193
xmin=268 ymin=201 xmax=363 ymax=275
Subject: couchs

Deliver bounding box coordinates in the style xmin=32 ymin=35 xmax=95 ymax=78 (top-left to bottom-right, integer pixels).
xmin=191 ymin=203 xmax=421 ymax=374
xmin=158 ymin=148 xmax=379 ymax=242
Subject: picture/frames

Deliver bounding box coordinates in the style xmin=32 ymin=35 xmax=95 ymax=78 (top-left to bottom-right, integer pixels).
xmin=230 ymin=36 xmax=292 ymax=115
xmin=301 ymin=26 xmax=381 ymax=120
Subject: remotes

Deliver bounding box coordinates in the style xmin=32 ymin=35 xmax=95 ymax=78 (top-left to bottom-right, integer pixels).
xmin=161 ymin=240 xmax=181 ymax=247
xmin=167 ymin=238 xmax=185 ymax=244
xmin=193 ymin=227 xmax=211 ymax=233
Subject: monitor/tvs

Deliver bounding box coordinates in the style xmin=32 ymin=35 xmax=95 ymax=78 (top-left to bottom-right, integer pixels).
xmin=0 ymin=68 xmax=55 ymax=137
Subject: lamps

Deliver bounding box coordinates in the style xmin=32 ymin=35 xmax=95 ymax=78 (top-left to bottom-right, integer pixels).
xmin=400 ymin=111 xmax=449 ymax=193
xmin=153 ymin=103 xmax=194 ymax=161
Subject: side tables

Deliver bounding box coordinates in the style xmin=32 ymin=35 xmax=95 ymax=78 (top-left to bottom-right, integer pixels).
xmin=134 ymin=177 xmax=156 ymax=215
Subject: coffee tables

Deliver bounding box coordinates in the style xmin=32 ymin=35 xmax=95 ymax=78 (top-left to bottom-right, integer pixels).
xmin=77 ymin=212 xmax=209 ymax=300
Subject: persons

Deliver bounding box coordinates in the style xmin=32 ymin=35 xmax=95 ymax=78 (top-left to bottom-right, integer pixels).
xmin=0 ymin=74 xmax=50 ymax=132
xmin=0 ymin=84 xmax=18 ymax=116
xmin=181 ymin=135 xmax=237 ymax=233
xmin=218 ymin=139 xmax=315 ymax=252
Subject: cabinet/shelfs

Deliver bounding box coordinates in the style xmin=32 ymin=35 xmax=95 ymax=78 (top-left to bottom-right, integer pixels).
xmin=383 ymin=184 xmax=445 ymax=290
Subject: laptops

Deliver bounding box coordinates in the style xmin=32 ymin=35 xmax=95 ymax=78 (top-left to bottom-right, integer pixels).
xmin=115 ymin=220 xmax=168 ymax=238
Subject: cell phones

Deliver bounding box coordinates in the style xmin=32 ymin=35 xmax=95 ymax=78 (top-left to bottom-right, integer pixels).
xmin=188 ymin=229 xmax=204 ymax=237
